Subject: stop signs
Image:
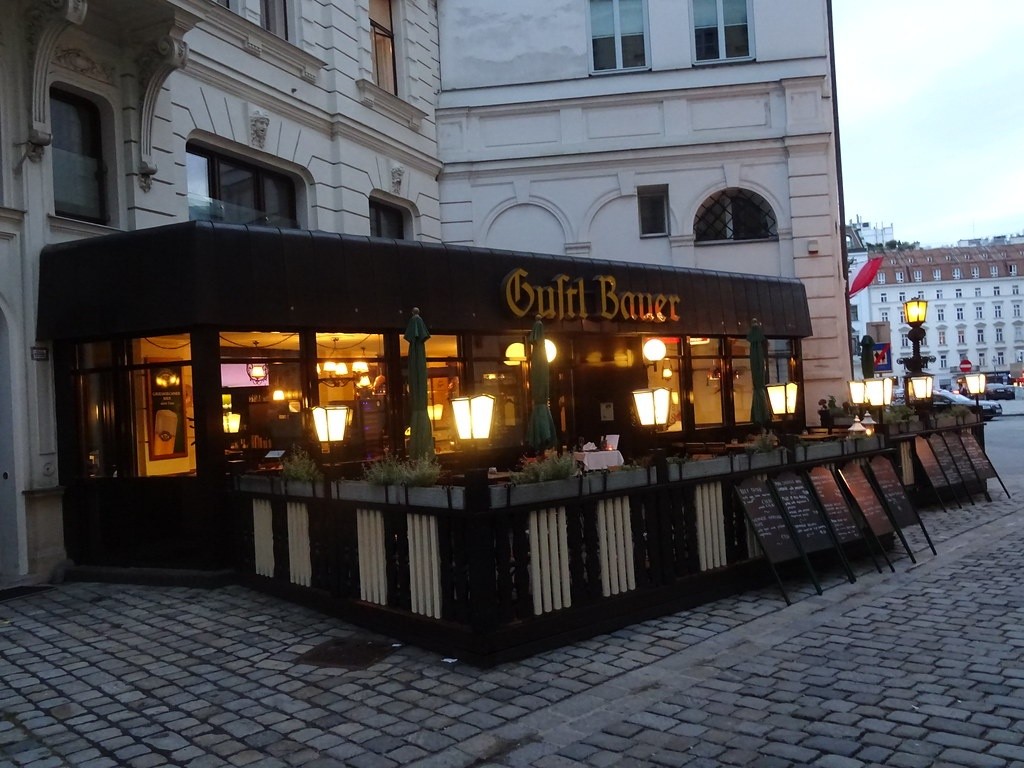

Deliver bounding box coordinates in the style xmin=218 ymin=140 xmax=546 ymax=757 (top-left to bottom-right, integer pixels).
xmin=960 ymin=360 xmax=972 ymax=372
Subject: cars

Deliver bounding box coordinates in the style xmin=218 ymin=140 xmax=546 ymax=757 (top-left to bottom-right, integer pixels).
xmin=978 ymin=383 xmax=1014 ymax=401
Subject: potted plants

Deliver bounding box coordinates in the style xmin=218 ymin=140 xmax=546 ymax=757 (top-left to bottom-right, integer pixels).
xmin=662 ymin=453 xmax=731 ymax=482
xmin=273 ymin=442 xmax=329 ymax=498
xmin=792 ymin=438 xmax=848 ymax=464
xmin=929 ymin=405 xmax=958 ymax=429
xmin=843 ymin=430 xmax=885 ymax=455
xmin=733 ymin=430 xmax=789 ymax=473
xmin=955 ymin=405 xmax=981 ymax=424
xmin=487 ymin=446 xmax=589 ymax=508
xmin=874 ymin=403 xmax=924 ymax=437
xmin=338 ymin=448 xmax=471 ymax=510
xmin=590 ymin=459 xmax=658 ymax=494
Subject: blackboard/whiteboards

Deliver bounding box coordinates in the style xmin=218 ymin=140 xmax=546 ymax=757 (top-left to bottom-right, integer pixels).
xmin=914 ymin=431 xmax=999 ymax=489
xmin=735 ymin=453 xmax=923 ymax=563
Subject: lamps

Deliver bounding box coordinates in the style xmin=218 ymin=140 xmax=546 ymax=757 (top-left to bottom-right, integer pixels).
xmin=314 ymin=338 xmax=372 ymax=389
xmin=708 ymin=359 xmax=749 ymax=382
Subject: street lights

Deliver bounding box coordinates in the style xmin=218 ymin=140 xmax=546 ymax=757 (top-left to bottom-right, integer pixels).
xmin=898 ymin=297 xmax=936 ymax=377
xmin=992 ymin=356 xmax=998 ymax=376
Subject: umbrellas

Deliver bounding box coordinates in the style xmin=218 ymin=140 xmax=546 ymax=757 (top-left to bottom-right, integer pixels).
xmin=404 ymin=305 xmax=436 ymax=467
xmin=747 ymin=318 xmax=770 ymax=441
xmin=525 ymin=313 xmax=557 ymax=455
xmin=861 ymin=335 xmax=876 ymax=380
